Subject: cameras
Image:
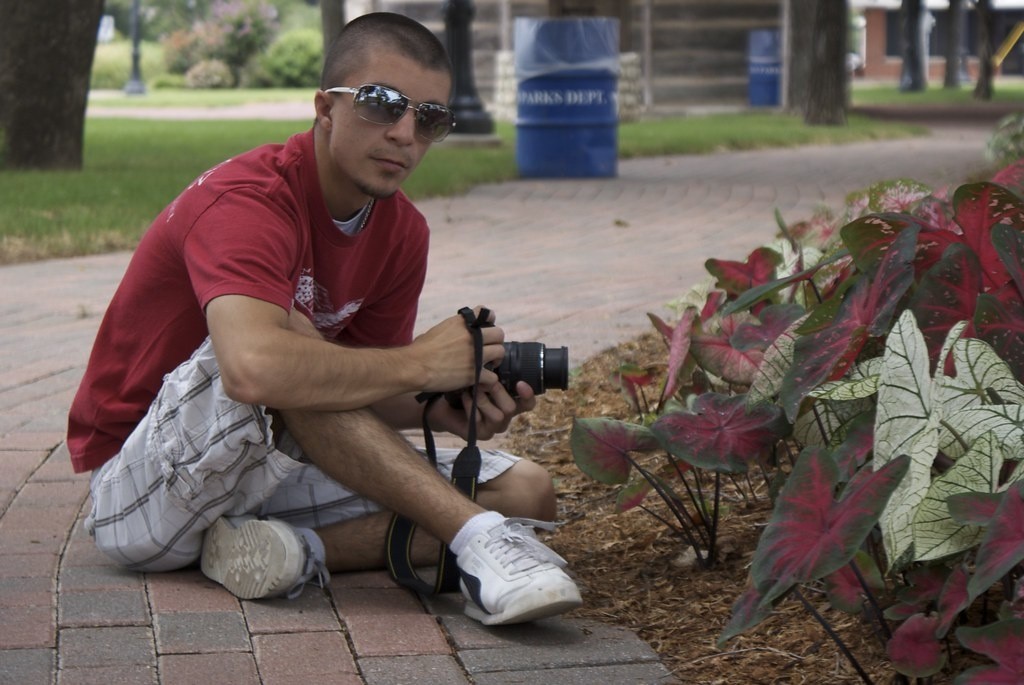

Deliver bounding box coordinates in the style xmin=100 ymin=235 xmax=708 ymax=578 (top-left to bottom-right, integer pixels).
xmin=444 ymin=339 xmax=570 ymax=410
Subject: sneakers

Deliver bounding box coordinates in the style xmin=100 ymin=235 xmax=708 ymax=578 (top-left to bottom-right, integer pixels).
xmin=201 ymin=513 xmax=331 ymax=600
xmin=456 ymin=516 xmax=583 ymax=626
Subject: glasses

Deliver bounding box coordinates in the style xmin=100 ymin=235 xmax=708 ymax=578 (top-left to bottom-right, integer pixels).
xmin=324 ymin=83 xmax=456 ymax=142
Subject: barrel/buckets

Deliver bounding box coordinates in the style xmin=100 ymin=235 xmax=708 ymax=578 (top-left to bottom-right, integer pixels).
xmin=513 ymin=17 xmax=619 ymax=178
xmin=748 ymin=29 xmax=781 ymax=104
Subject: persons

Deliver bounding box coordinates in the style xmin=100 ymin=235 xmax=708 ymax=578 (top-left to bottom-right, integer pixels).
xmin=66 ymin=12 xmax=581 ymax=627
